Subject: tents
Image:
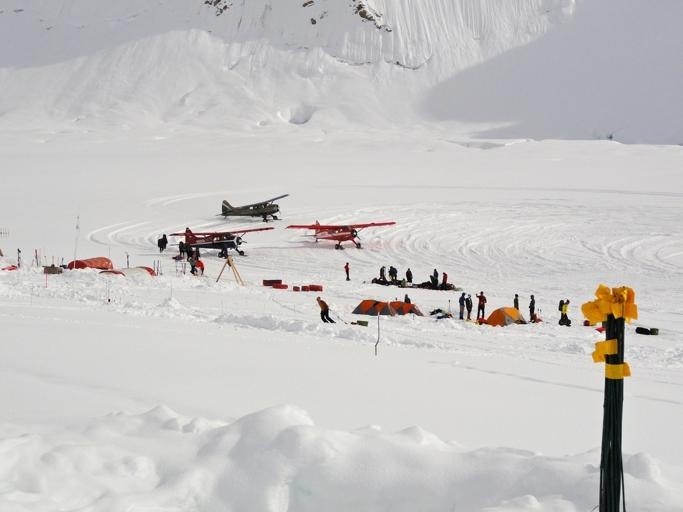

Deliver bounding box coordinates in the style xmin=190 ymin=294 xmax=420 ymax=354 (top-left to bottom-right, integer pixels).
xmin=68 ymin=257 xmax=113 ymax=271
xmin=486 ymin=306 xmax=526 ymax=327
xmin=99 ymin=266 xmax=157 ymax=278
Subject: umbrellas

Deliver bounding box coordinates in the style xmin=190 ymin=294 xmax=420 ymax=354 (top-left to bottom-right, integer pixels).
xmin=352 ymin=300 xmax=423 ymax=315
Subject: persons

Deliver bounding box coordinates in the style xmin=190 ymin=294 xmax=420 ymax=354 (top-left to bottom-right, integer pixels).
xmin=406 ymin=268 xmax=413 ymax=283
xmin=476 ymin=291 xmax=487 ymax=320
xmin=379 ymin=266 xmax=386 ymax=280
xmin=158 ymin=234 xmax=167 ymax=252
xmin=389 ymin=266 xmax=397 ymax=280
xmin=179 ymin=241 xmax=204 ymax=275
xmin=405 ymin=294 xmax=411 ymax=303
xmin=317 ymin=296 xmax=336 ymax=323
xmin=459 ymin=293 xmax=466 ymax=319
xmin=430 ymin=269 xmax=438 ymax=287
xmin=442 ymin=272 xmax=447 ymax=288
xmin=529 ymin=295 xmax=535 ymax=323
xmin=344 ymin=262 xmax=350 ymax=281
xmin=466 ymin=294 xmax=472 ymax=320
xmin=559 ymin=300 xmax=569 ymax=321
xmin=514 ymin=294 xmax=519 ymax=310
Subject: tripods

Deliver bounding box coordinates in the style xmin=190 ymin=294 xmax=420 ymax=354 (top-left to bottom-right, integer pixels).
xmin=216 ymin=255 xmax=244 ymax=286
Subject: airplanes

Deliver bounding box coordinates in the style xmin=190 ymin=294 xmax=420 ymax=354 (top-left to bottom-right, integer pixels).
xmin=169 ymin=227 xmax=275 ymax=257
xmin=287 ymin=221 xmax=396 ymax=249
xmin=216 ymin=194 xmax=290 ymax=221
